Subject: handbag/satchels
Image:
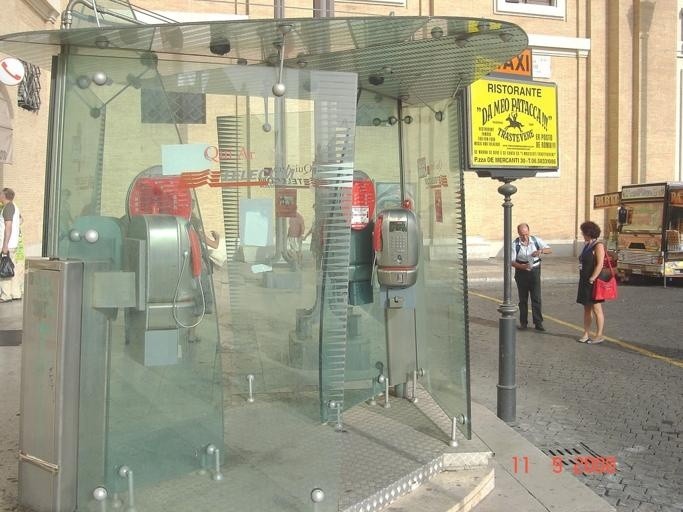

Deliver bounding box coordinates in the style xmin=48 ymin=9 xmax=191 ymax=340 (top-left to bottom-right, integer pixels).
xmin=0 ymin=251 xmax=14 ymax=278
xmin=592 ymin=276 xmax=618 ymax=301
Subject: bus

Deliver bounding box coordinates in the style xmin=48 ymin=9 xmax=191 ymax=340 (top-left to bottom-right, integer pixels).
xmin=616 ymin=182 xmax=683 ymax=285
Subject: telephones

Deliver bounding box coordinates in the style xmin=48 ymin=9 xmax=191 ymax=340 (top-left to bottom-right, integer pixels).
xmin=127 ymin=212 xmax=202 ymax=330
xmin=374 ymin=209 xmax=422 ymax=287
xmin=329 ymin=171 xmax=375 ymax=266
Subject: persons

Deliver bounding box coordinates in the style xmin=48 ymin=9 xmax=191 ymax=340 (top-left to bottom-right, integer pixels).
xmin=509 ymin=223 xmax=552 ymax=332
xmin=301 ymin=204 xmax=317 ymax=261
xmin=284 ymin=211 xmax=305 ymax=262
xmin=0 ymin=187 xmax=24 ymax=303
xmin=574 ymin=222 xmax=608 ymax=344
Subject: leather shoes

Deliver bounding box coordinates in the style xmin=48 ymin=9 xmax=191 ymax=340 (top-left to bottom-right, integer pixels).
xmin=518 ymin=324 xmax=527 ymax=329
xmin=587 ymin=338 xmax=604 ymax=344
xmin=578 ymin=335 xmax=589 ymax=343
xmin=535 ymin=324 xmax=545 ymax=331
